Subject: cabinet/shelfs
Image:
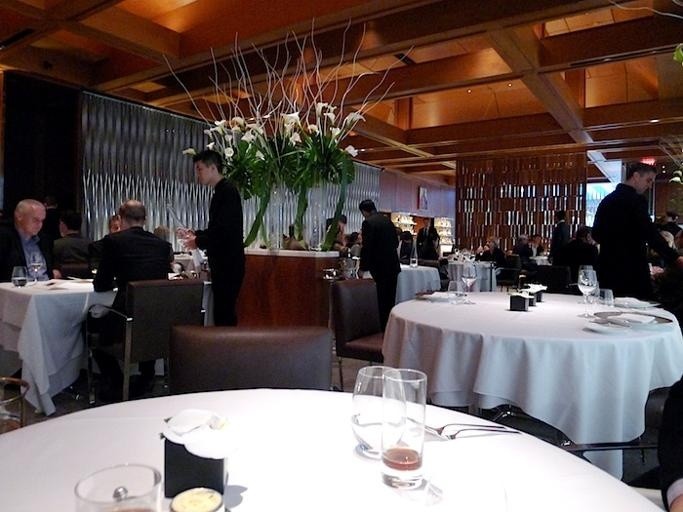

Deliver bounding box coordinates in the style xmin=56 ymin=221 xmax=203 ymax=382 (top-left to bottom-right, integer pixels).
xmin=434 ymin=216 xmax=455 ymax=258
xmin=392 ymin=211 xmax=417 ymax=237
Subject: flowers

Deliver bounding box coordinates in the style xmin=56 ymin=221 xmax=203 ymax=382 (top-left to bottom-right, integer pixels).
xmin=169 ymin=40 xmax=356 ymax=252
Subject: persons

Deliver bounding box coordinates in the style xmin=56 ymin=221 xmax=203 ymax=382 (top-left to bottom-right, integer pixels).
xmin=108 ymin=213 xmax=120 ymax=234
xmin=79 ymin=200 xmax=173 ymax=401
xmin=327 ymin=214 xmax=440 ymax=264
xmin=591 ymin=162 xmax=683 ymax=297
xmin=550 ymin=211 xmax=681 ymax=282
xmin=630 ymin=375 xmax=683 ymax=512
xmin=476 ymin=233 xmax=544 ymax=278
xmin=52 ymin=210 xmax=92 ymax=261
xmin=177 ymin=150 xmax=245 ymax=327
xmin=0 ymin=198 xmax=62 ymax=281
xmin=360 ymin=199 xmax=402 ymax=331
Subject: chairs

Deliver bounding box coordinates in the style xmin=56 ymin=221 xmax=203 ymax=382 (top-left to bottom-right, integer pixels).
xmin=161 ymin=324 xmax=337 ymax=397
xmin=501 ymin=255 xmax=526 ymax=293
xmin=332 ymin=278 xmax=387 ymax=389
xmin=88 ymin=276 xmax=205 ymax=404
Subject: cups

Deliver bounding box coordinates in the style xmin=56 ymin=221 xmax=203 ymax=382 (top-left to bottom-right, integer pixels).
xmin=74 ymin=461 xmax=162 ymax=512
xmin=540 ymin=251 xmax=549 ymax=257
xmin=598 ymin=289 xmax=615 ymax=308
xmin=169 ymin=487 xmax=225 ymax=512
xmin=447 ymin=279 xmax=461 ymax=304
xmin=409 ymin=258 xmax=418 ymax=268
xmin=350 ymin=365 xmax=408 ymax=456
xmin=11 ymin=266 xmax=28 ymax=287
xmin=380 ymin=368 xmax=428 ymax=489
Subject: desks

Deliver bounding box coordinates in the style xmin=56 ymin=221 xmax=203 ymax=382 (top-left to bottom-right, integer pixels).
xmin=0 ymin=273 xmax=112 ymax=412
xmin=530 ymin=256 xmax=553 ymax=267
xmin=396 ymin=265 xmax=441 ymax=306
xmin=449 ymin=256 xmax=495 ymax=295
xmin=383 ymin=290 xmax=682 ymax=479
xmin=0 ymin=386 xmax=662 ymax=506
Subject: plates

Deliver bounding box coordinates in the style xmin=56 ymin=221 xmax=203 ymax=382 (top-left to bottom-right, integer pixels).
xmin=607 ymin=298 xmax=661 ymax=309
xmin=582 ymin=318 xmax=631 ymax=334
xmin=594 ymin=308 xmax=674 ymax=328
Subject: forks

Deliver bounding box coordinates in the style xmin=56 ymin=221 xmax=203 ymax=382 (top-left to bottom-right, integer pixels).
xmin=424 ymin=427 xmax=519 ymax=442
xmin=410 ymin=419 xmax=503 ymax=435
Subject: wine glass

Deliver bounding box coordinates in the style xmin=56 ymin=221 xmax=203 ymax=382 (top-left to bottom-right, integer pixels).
xmin=452 ymin=246 xmax=475 ymax=262
xmin=29 ymin=250 xmax=45 ymax=287
xmin=577 ymin=265 xmax=597 ymax=320
xmin=460 ymin=262 xmax=478 ymax=305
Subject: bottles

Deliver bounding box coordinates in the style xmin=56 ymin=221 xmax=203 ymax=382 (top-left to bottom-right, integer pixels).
xmin=164 ymin=406 xmax=229 ymax=498
xmin=648 ymin=263 xmax=653 ymax=274
